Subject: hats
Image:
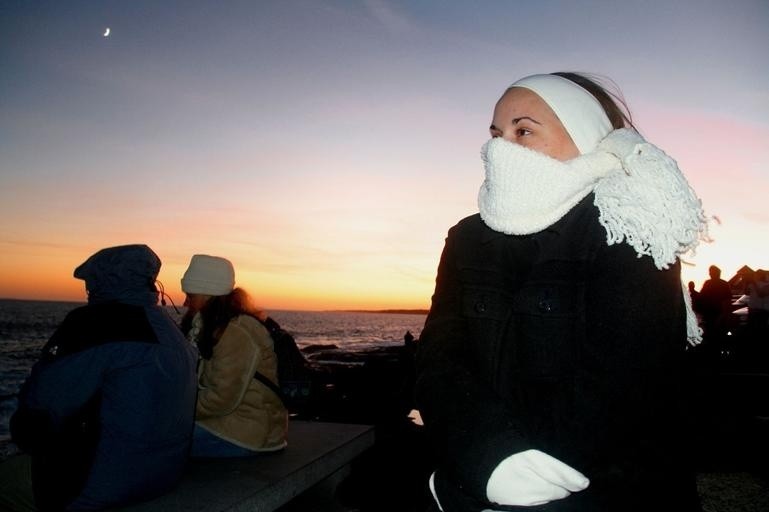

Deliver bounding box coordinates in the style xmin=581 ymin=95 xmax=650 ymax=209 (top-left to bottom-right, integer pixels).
xmin=181 ymin=254 xmax=235 ymax=296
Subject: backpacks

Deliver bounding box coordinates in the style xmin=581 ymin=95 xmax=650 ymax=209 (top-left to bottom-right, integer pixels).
xmin=220 ymin=311 xmax=327 ymax=413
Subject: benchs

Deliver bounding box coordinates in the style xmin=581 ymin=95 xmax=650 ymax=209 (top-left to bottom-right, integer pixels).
xmin=162 ymin=421 xmax=375 ymax=512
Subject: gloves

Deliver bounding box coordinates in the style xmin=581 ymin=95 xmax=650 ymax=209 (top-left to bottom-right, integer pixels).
xmin=486 ymin=448 xmax=591 ymax=506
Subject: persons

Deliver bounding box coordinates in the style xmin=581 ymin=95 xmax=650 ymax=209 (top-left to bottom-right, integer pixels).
xmin=736 ymin=269 xmax=769 ymax=322
xmin=688 ymin=281 xmax=700 ymax=318
xmin=179 ymin=256 xmax=290 ymax=464
xmin=9 ymin=246 xmax=198 ymax=512
xmin=700 ymin=265 xmax=731 ymax=346
xmin=412 ymin=72 xmax=702 ymax=512
